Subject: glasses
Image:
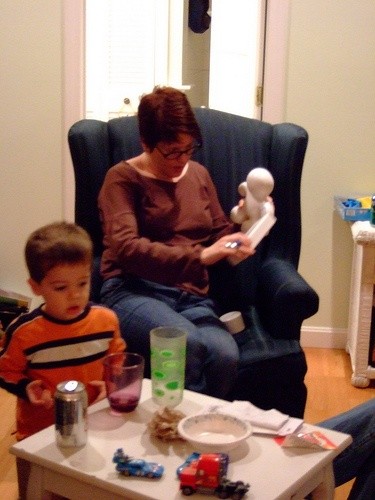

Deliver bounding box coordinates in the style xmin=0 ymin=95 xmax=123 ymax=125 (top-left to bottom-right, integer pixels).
xmin=156 ymin=142 xmax=202 ymax=161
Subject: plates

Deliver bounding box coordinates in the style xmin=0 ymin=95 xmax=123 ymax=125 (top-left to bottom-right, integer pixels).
xmin=176 ymin=412 xmax=254 ymax=454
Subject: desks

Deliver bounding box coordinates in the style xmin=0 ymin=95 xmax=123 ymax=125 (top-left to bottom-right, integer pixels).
xmin=9 ymin=377 xmax=352 ymax=500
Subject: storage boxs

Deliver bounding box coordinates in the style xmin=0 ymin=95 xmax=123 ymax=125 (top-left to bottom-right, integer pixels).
xmin=338 ymin=199 xmax=372 ymax=220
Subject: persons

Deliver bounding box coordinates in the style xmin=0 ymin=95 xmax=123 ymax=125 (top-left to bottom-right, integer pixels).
xmin=304 ymin=397 xmax=375 ymax=500
xmin=0 ymin=221 xmax=125 ymax=500
xmin=99 ymin=86 xmax=256 ymax=397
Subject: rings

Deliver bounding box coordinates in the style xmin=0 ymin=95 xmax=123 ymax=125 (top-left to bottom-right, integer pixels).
xmin=225 ymin=242 xmax=237 ymax=248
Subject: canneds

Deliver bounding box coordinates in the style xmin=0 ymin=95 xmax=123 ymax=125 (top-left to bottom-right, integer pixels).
xmin=53 ymin=380 xmax=87 ymax=447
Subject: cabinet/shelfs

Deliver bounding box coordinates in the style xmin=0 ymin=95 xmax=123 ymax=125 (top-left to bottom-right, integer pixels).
xmin=344 ymin=222 xmax=375 ymax=388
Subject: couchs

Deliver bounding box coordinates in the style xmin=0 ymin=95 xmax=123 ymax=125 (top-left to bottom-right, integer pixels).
xmin=67 ymin=107 xmax=319 ymax=418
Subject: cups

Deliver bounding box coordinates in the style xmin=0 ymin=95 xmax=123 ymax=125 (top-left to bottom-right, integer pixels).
xmin=150 ymin=327 xmax=187 ymax=409
xmin=101 ymin=352 xmax=145 ymax=414
